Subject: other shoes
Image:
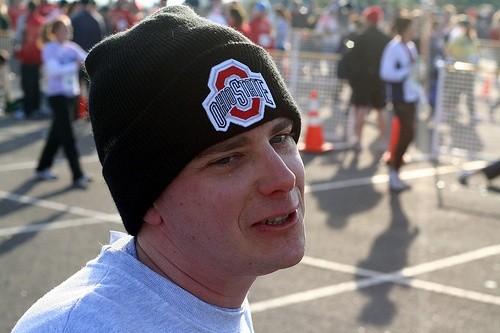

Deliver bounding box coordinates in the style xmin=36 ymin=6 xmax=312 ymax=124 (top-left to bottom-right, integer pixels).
xmin=36 ymin=169 xmax=58 ymax=178
xmin=389 ymin=177 xmax=411 ymax=191
xmin=76 ymin=175 xmax=92 ymax=186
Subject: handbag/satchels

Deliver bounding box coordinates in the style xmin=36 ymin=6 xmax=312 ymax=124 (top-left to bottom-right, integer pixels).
xmin=415 ymin=85 xmax=431 ymax=121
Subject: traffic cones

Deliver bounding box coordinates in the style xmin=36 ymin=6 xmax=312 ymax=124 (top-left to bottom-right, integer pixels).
xmin=302 ymin=91 xmax=328 ymax=155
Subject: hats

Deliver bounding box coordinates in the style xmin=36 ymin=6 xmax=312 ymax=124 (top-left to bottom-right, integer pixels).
xmin=364 ymin=6 xmax=382 ymax=23
xmin=85 ymin=5 xmax=301 ymax=236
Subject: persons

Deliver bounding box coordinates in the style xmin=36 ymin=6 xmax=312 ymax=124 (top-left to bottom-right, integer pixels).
xmin=10 ymin=5 xmax=306 ymax=333
xmin=0 ymin=0 xmax=500 ymax=193
